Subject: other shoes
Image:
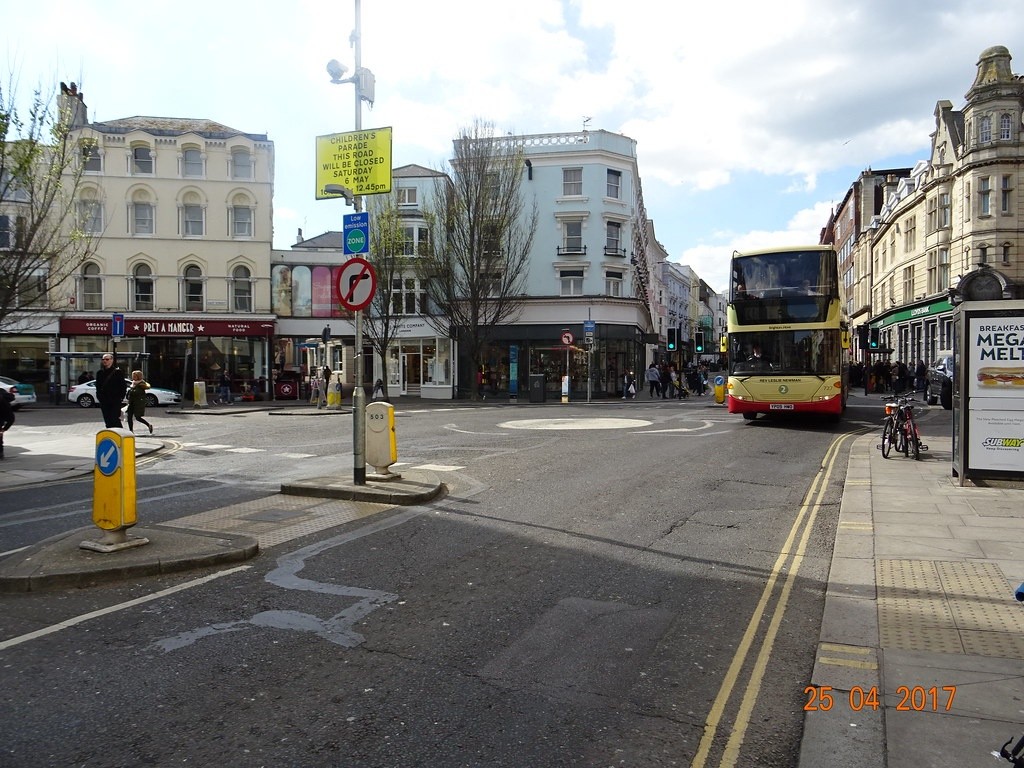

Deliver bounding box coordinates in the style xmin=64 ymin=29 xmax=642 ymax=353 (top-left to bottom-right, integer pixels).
xmin=213 ymin=400 xmax=217 ymax=405
xmin=149 ymin=425 xmax=153 ymax=433
xmin=226 ymin=402 xmax=234 ymax=405
xmin=621 ymin=396 xmax=626 ymax=399
xmin=482 ymin=396 xmax=486 ymax=401
xmin=632 ymin=395 xmax=635 ymax=398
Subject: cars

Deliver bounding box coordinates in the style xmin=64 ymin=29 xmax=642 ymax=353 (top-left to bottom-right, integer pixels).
xmin=69 ymin=378 xmax=182 ymax=407
xmin=0 ymin=376 xmax=36 ymax=410
xmin=924 ymin=355 xmax=953 ymax=410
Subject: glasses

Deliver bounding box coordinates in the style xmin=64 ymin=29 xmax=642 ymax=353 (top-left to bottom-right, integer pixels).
xmin=102 ymin=359 xmax=109 ymax=361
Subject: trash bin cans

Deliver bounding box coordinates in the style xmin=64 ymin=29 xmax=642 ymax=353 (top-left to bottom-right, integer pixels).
xmin=530 ymin=374 xmax=547 ymax=403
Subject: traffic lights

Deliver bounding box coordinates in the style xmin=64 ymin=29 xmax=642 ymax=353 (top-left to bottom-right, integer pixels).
xmin=694 ymin=332 xmax=704 ymax=353
xmin=666 ymin=327 xmax=677 ymax=351
xmin=870 ymin=327 xmax=879 ymax=346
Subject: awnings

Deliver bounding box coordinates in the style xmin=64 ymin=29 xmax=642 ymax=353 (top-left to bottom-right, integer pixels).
xmin=60 ymin=312 xmax=276 ymax=337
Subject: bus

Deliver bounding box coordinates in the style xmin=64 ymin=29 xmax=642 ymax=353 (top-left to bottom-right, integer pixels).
xmin=720 ymin=241 xmax=849 ymax=422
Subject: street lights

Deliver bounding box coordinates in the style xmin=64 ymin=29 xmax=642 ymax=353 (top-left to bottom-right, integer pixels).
xmin=325 ymin=184 xmax=365 ymax=486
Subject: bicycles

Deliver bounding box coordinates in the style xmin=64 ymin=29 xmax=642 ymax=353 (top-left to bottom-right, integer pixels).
xmin=877 ymin=391 xmax=928 ymax=461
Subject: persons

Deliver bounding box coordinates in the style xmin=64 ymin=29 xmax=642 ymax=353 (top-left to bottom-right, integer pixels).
xmin=716 ymin=356 xmax=728 ymax=371
xmin=849 ymin=359 xmax=926 ymax=394
xmin=478 ymin=367 xmax=486 ymax=401
xmin=323 ymin=366 xmax=331 ymax=385
xmin=621 ymin=369 xmax=635 ymax=400
xmin=96 ymin=354 xmax=127 ymax=428
xmin=749 ymin=344 xmax=772 ymax=371
xmin=390 ymin=354 xmax=398 ymax=384
xmin=213 ymin=369 xmax=234 ymax=405
xmin=0 ymin=388 xmax=15 ymax=461
xmin=646 ymin=363 xmax=684 ymax=399
xmin=126 ymin=370 xmax=153 ymax=434
xmin=78 ymin=370 xmax=94 ymax=384
xmin=687 ymin=360 xmax=708 ymax=396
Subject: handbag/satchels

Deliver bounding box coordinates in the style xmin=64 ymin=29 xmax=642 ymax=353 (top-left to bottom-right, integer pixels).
xmin=628 ymin=384 xmax=636 ymax=393
